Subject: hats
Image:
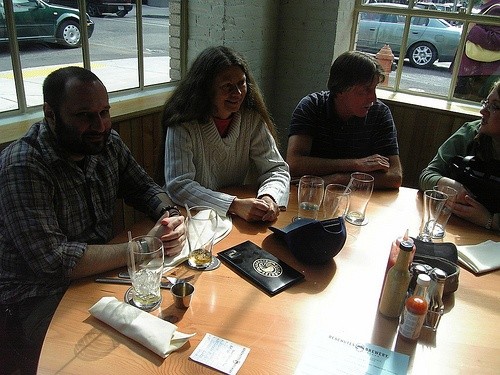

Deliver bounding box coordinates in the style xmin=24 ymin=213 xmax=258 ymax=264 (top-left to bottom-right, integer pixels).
xmin=266 ymin=216 xmax=348 ymax=267
xmin=407 ymin=236 xmax=460 ymax=295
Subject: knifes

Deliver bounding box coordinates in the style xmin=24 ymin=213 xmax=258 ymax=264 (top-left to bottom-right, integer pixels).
xmin=94 ymin=277 xmax=173 ymax=289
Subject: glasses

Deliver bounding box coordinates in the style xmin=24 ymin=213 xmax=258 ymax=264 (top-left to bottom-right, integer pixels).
xmin=480 ymin=100 xmax=500 ymax=113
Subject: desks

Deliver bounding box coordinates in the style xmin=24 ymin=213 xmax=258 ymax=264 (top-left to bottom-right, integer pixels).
xmin=37 ymin=187 xmax=500 ymax=375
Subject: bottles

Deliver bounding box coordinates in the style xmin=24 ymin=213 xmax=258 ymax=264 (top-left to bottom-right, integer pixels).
xmin=398 ymin=274 xmax=431 ymax=344
xmin=378 ymin=229 xmax=416 ymax=301
xmin=378 ymin=241 xmax=414 ymax=319
xmin=422 ymin=269 xmax=447 ymax=331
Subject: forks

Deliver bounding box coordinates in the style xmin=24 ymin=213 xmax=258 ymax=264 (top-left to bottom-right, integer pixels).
xmin=119 ymin=273 xmax=194 ymax=285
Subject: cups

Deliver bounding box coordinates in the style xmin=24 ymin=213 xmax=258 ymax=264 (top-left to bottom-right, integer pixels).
xmin=345 ymin=172 xmax=375 ymax=222
xmin=127 ymin=235 xmax=164 ymax=310
xmin=423 ymin=185 xmax=457 ymax=239
xmin=416 ymin=190 xmax=449 ymax=243
xmin=171 ymin=283 xmax=194 ymax=309
xmin=298 ymin=175 xmax=324 ymax=219
xmin=184 ymin=206 xmax=218 ymax=269
xmin=324 ymin=184 xmax=352 ymax=219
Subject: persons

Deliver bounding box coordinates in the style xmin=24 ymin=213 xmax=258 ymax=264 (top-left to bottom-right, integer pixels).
xmin=161 ymin=46 xmax=291 ymax=226
xmin=448 ymin=0 xmax=500 ymax=102
xmin=419 ymin=79 xmax=500 ymax=231
xmin=286 ymin=50 xmax=402 ymax=188
xmin=361 ymin=0 xmax=380 ymax=21
xmin=0 ymin=67 xmax=187 ymax=375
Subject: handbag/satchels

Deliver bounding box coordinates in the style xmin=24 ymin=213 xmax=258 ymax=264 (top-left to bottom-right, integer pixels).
xmin=444 ymin=153 xmax=500 ymax=214
xmin=465 ymin=3 xmax=500 ymax=63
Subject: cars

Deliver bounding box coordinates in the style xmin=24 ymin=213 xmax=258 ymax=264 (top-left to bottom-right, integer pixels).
xmin=355 ymin=3 xmax=463 ymax=69
xmin=0 ymin=0 xmax=95 ymax=49
xmin=41 ymin=0 xmax=133 ymax=18
xmin=414 ymin=2 xmax=465 ymax=10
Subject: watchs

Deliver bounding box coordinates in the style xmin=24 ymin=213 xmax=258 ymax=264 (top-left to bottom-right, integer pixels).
xmin=484 ymin=212 xmax=493 ymax=229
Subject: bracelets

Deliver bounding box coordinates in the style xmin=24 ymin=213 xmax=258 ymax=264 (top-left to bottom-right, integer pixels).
xmin=160 ymin=206 xmax=182 ymax=216
xmin=138 ymin=239 xmax=150 ymax=260
xmin=135 ymin=240 xmax=143 ymax=263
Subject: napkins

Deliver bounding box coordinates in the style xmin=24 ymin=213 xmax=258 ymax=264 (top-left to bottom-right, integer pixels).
xmin=457 ymin=240 xmax=500 ymax=273
xmin=88 ymin=297 xmax=196 ymax=359
xmin=145 ymin=211 xmax=233 ymax=274
